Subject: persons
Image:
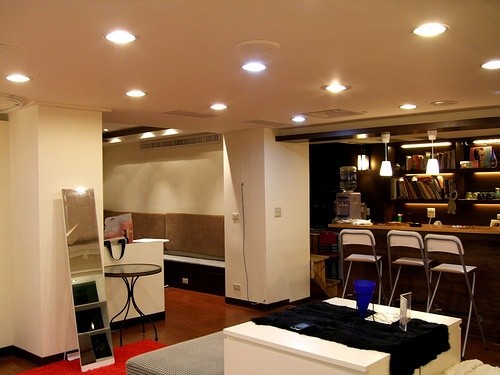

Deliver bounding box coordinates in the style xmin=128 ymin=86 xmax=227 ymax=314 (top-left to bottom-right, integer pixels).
xmin=474 ymin=147 xmax=485 ymax=168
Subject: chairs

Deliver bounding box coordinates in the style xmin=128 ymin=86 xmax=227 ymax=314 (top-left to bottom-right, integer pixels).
xmin=317 ymin=231 xmax=340 ymax=280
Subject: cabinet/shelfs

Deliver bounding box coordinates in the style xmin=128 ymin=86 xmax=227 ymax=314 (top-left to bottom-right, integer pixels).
xmin=396 ymin=139 xmax=500 ymax=201
xmin=311 ymin=253 xmax=341 ymax=298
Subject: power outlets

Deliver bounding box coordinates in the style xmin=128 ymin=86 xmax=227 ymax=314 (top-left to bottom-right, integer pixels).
xmin=427 ymin=208 xmax=435 ymax=218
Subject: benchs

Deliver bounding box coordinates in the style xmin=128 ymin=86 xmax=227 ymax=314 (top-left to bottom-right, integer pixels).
xmin=103 ymin=210 xmax=225 ymax=269
xmin=125 ymin=330 xmax=224 ymax=375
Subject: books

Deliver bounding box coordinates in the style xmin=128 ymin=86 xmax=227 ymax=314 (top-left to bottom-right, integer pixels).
xmin=408 ymin=150 xmax=455 ymax=170
xmin=394 ymin=177 xmax=455 ymax=200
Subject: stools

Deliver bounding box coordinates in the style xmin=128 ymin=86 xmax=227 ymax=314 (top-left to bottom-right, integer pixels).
xmin=386 ymin=230 xmax=439 ymax=313
xmin=423 ymin=232 xmax=490 ymax=360
xmin=339 ymin=228 xmax=388 ymax=306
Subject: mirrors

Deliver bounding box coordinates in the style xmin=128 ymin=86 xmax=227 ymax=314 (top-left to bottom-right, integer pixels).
xmin=62 ymin=186 xmax=118 ymax=371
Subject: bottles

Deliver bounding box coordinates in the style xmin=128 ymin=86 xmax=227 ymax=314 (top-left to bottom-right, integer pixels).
xmin=340 ymin=166 xmax=357 ymax=192
xmin=489 ymin=149 xmax=497 ymax=168
xmin=495 ymin=188 xmax=500 ymax=199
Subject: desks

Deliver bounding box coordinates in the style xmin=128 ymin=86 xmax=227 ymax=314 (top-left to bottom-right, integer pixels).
xmin=104 ymin=263 xmax=163 ymax=346
xmin=222 ymin=297 xmax=464 ymax=375
xmin=103 ymin=236 xmax=171 ymax=328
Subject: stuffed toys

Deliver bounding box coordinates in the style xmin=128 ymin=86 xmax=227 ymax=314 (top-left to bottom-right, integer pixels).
xmin=444 ymin=190 xmax=457 ymax=214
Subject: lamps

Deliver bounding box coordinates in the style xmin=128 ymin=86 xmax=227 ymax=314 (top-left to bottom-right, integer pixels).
xmin=380 ymin=132 xmax=393 ymax=177
xmin=426 ymin=130 xmax=439 ymax=176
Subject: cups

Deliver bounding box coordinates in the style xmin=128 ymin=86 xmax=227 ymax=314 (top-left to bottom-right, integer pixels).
xmin=466 ymin=192 xmax=496 ymax=199
xmin=434 ymin=220 xmax=441 ymax=226
xmin=353 ymin=280 xmax=377 ymax=319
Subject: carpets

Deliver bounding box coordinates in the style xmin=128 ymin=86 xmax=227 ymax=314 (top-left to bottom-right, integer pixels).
xmin=17 ymin=338 xmax=168 ymax=375
